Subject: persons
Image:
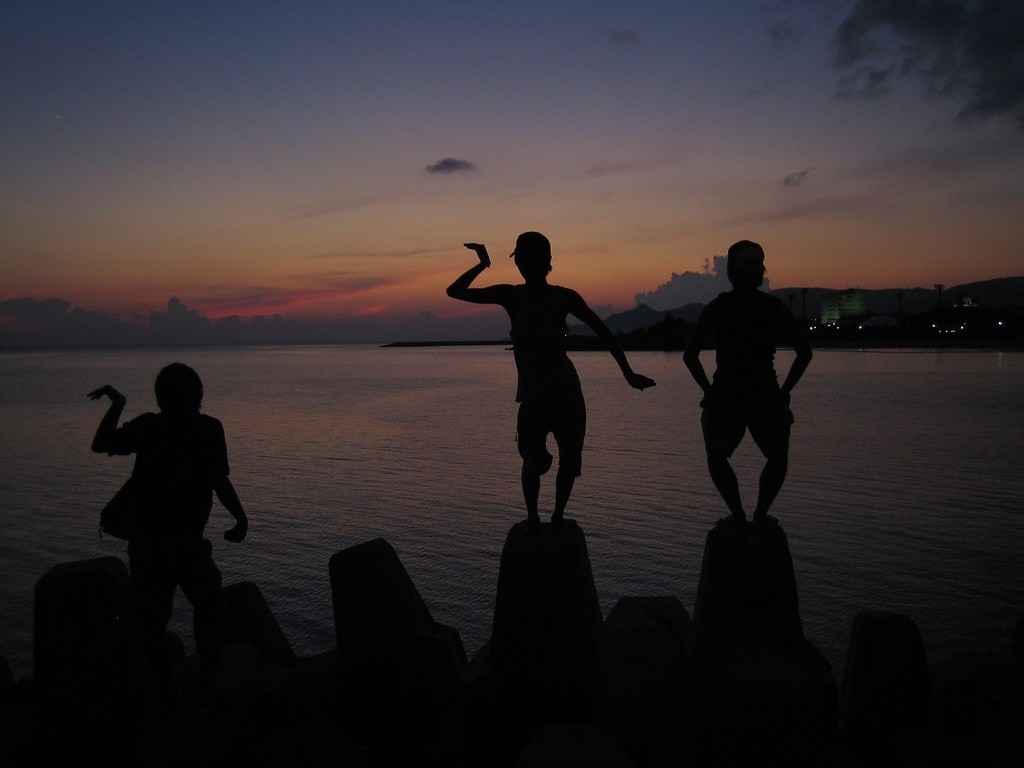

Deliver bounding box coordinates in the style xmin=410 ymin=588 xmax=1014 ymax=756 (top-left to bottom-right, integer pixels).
xmin=87 ymin=363 xmax=248 ymax=659
xmin=683 ymin=241 xmax=813 ymax=536
xmin=446 ymin=232 xmax=656 ymax=528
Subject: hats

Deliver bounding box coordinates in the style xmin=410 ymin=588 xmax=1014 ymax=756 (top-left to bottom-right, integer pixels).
xmin=511 ymin=232 xmax=549 ymax=259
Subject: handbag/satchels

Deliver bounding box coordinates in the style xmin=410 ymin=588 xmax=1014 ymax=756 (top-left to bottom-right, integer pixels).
xmin=100 ymin=487 xmax=145 ymax=540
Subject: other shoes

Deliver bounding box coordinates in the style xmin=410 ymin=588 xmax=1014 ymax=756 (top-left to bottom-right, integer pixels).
xmin=528 ymin=512 xmax=540 ymax=530
xmin=751 ymin=516 xmax=779 ymax=536
xmin=551 ymin=516 xmax=576 ymax=536
xmin=716 ymin=513 xmax=746 ymax=535
